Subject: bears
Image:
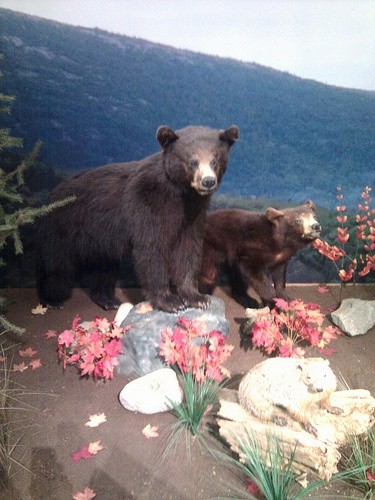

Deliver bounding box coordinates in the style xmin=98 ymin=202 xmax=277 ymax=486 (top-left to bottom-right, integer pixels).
xmin=31 ymin=124 xmax=321 ymax=317
